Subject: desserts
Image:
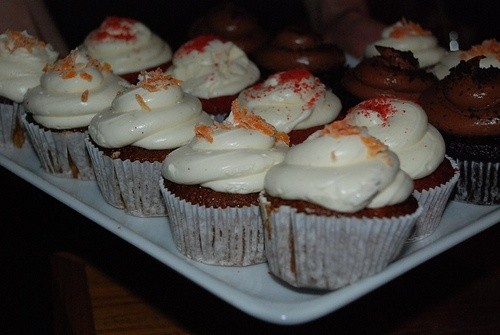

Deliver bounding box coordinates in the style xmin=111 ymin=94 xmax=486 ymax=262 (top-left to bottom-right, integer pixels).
xmin=0 ymin=0 xmax=500 ymax=292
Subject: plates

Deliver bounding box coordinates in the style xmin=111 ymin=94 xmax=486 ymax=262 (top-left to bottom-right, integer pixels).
xmin=0 ymin=127 xmax=500 ymax=325
